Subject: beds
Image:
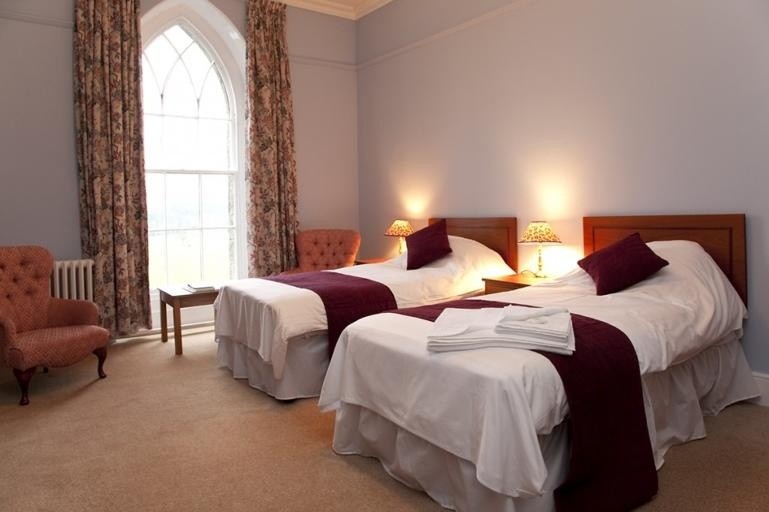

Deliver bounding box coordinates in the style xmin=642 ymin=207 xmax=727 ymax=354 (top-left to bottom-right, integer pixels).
xmin=318 ymin=212 xmax=746 ymax=511
xmin=219 ymin=216 xmax=518 ymax=403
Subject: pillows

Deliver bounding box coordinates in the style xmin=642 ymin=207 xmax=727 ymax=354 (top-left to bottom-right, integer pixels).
xmin=576 ymin=233 xmax=670 ymax=296
xmin=404 ymin=218 xmax=452 ymax=270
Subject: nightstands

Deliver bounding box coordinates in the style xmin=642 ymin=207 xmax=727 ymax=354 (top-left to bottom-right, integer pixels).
xmin=481 ymin=272 xmax=532 ymax=293
xmin=354 ymin=257 xmax=384 ymax=264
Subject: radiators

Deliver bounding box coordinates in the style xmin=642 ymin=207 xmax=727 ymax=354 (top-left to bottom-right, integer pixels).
xmin=50 ymin=259 xmax=94 ymax=303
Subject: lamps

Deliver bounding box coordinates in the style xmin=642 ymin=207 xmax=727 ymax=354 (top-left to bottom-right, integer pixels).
xmin=384 ymin=220 xmax=415 ymax=254
xmin=516 ymin=222 xmax=563 ymax=280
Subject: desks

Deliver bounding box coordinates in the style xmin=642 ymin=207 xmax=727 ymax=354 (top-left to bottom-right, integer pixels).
xmin=158 ymin=286 xmax=220 ymax=355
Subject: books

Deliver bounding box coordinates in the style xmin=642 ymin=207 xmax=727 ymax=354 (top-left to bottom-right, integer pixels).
xmin=182 ymin=285 xmax=216 ymax=293
xmin=188 ymin=283 xmax=214 ymax=289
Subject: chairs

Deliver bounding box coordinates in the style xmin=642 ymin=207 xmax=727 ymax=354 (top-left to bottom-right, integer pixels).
xmin=0 ymin=244 xmax=110 ymax=406
xmin=276 ymin=229 xmax=361 ymax=277
